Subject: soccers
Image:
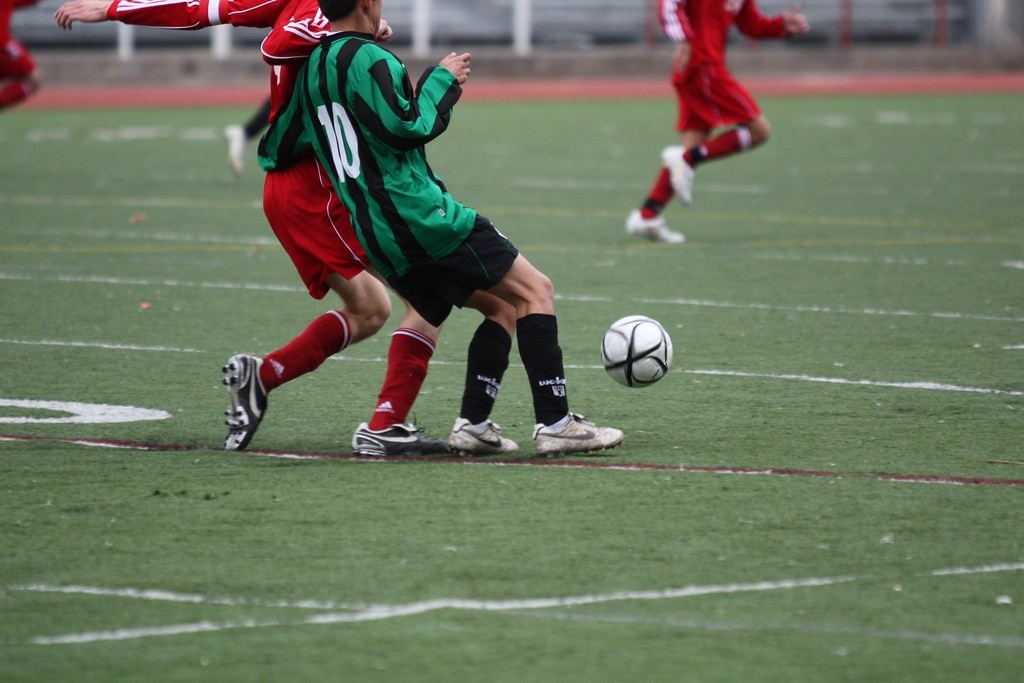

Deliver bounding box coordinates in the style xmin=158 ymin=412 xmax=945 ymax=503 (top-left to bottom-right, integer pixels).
xmin=600 ymin=314 xmax=675 ymax=389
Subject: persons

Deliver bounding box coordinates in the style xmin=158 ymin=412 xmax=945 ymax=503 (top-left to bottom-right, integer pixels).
xmin=53 ymin=0 xmax=464 ymax=458
xmin=0 ymin=0 xmax=43 ymax=108
xmin=257 ymin=0 xmax=625 ymax=458
xmin=625 ymin=1 xmax=810 ymax=243
xmin=225 ymin=94 xmax=272 ymax=177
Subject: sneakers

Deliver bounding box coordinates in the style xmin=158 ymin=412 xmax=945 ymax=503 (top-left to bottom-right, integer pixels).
xmin=625 ymin=210 xmax=685 ymax=245
xmin=533 ymin=412 xmax=625 ymax=457
xmin=222 ymin=354 xmax=268 ymax=451
xmin=663 ymin=145 xmax=695 ymax=205
xmin=450 ymin=417 xmax=519 ymax=455
xmin=351 ymin=413 xmax=460 ymax=457
